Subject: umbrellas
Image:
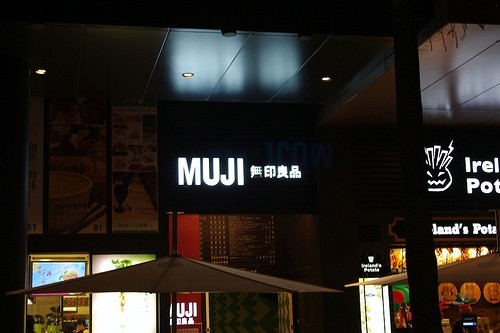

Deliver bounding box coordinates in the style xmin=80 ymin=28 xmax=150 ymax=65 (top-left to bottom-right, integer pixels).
xmin=6 ymin=251 xmax=345 ymax=333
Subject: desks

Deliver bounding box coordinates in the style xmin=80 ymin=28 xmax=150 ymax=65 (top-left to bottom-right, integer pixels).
xmin=112 ymin=178 xmax=157 ymax=231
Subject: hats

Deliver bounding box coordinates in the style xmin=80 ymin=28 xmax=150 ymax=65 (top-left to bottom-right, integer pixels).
xmin=73 ymin=318 xmax=86 ymax=325
